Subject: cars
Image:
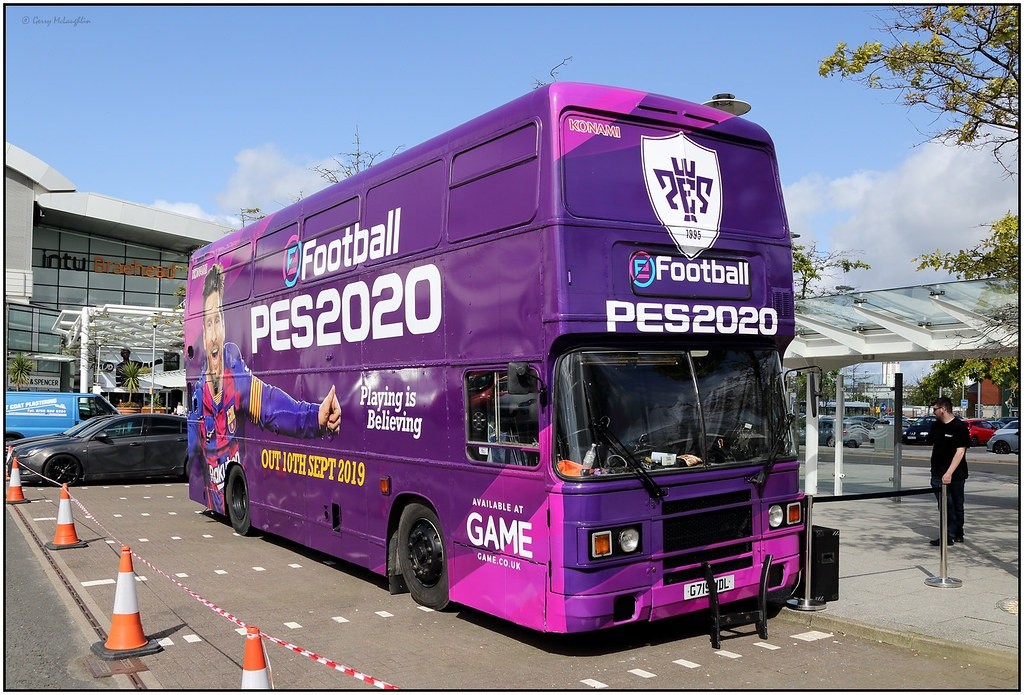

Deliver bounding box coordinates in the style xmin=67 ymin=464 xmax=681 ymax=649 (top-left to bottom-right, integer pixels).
xmin=795 ymin=414 xmax=1019 ymax=450
xmin=6 ymin=412 xmax=193 ymax=489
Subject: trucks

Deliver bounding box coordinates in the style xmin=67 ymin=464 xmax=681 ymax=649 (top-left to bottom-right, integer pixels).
xmin=6 ymin=390 xmax=121 ymax=443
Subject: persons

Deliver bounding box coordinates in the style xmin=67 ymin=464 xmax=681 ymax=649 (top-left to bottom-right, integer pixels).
xmin=925 ymin=398 xmax=971 ymax=546
xmin=190 ymin=266 xmax=341 ymax=518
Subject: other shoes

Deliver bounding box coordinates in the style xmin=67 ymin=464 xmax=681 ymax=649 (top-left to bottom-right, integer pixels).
xmin=955 ymin=534 xmax=964 ymax=542
xmin=930 ymin=536 xmax=954 ymax=545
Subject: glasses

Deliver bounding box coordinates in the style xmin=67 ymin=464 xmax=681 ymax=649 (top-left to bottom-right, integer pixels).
xmin=934 ymin=407 xmax=946 ymax=412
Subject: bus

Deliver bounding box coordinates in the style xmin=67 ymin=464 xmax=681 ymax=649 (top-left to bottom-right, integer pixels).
xmin=183 ymin=79 xmax=806 ymax=646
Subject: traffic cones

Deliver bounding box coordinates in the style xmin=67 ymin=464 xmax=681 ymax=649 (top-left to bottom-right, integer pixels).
xmin=44 ymin=482 xmax=89 ymax=550
xmin=6 ymin=445 xmax=13 ymax=464
xmin=6 ymin=456 xmax=31 ymax=505
xmin=89 ymin=545 xmax=166 ymax=662
xmin=240 ymin=626 xmax=273 ymax=689
